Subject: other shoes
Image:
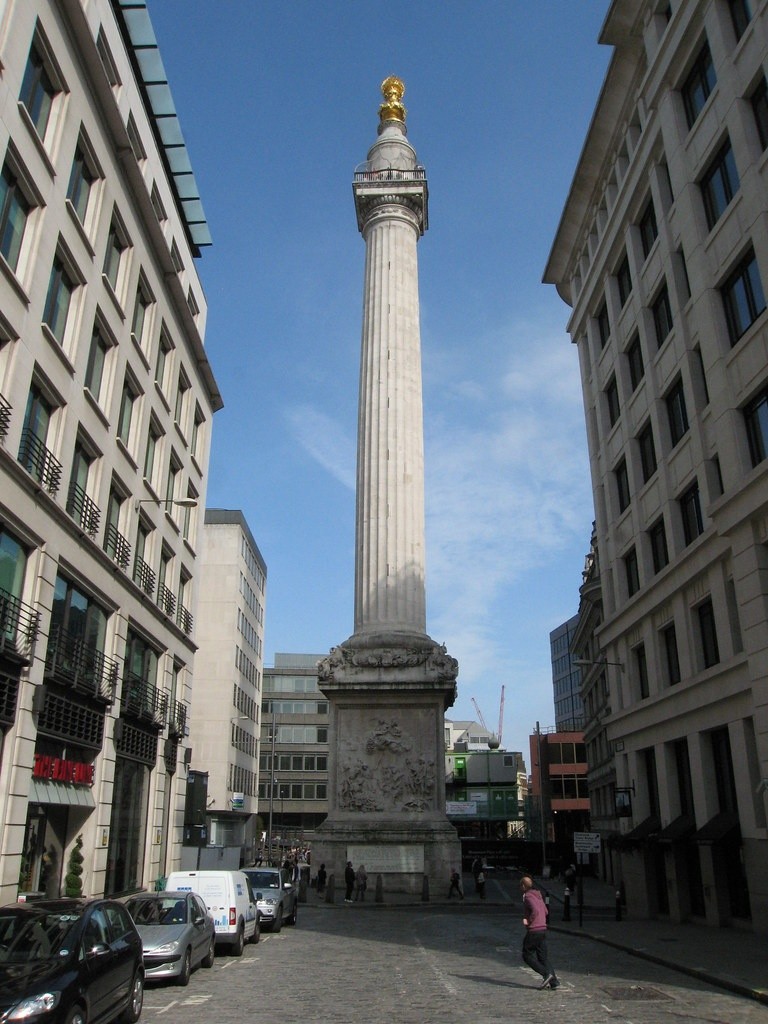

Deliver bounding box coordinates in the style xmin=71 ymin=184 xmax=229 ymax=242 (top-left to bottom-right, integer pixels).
xmin=539 ymin=974 xmax=554 ymax=990
xmin=553 ymin=984 xmax=560 ymax=990
xmin=344 ymin=898 xmax=354 ymax=903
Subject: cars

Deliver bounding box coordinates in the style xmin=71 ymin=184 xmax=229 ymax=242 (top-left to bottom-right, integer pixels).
xmin=103 ymin=891 xmax=216 ymax=985
xmin=239 ymin=866 xmax=300 ymax=932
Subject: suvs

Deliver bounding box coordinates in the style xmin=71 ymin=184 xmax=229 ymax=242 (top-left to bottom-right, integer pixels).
xmin=0 ymin=898 xmax=145 ymax=1024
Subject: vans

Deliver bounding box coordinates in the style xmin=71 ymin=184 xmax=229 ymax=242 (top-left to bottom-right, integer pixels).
xmin=166 ymin=871 xmax=264 ymax=956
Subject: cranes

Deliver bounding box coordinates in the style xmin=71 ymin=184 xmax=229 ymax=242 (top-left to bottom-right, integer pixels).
xmin=471 ymin=684 xmax=506 ymax=745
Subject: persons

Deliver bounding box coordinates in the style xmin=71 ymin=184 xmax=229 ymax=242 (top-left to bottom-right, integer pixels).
xmin=472 ymin=858 xmax=486 ymax=899
xmin=344 ymin=861 xmax=355 ymax=903
xmin=253 ymin=848 xmax=263 ymax=867
xmin=318 ymin=864 xmax=327 ymax=898
xmin=445 ymin=867 xmax=464 ymax=900
xmin=519 ymin=877 xmax=560 ymax=990
xmin=355 ymin=864 xmax=368 ymax=901
xmin=284 ymin=855 xmax=297 ymax=869
